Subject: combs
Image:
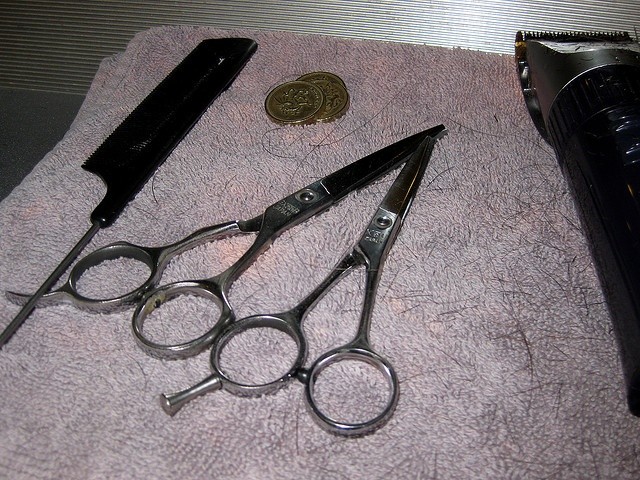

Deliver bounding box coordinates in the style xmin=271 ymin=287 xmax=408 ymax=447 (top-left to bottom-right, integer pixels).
xmin=0 ymin=37 xmax=259 ymax=348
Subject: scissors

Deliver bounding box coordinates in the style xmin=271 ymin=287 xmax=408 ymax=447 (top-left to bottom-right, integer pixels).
xmin=6 ymin=123 xmax=448 ymax=361
xmin=162 ymin=135 xmax=437 ymax=437
